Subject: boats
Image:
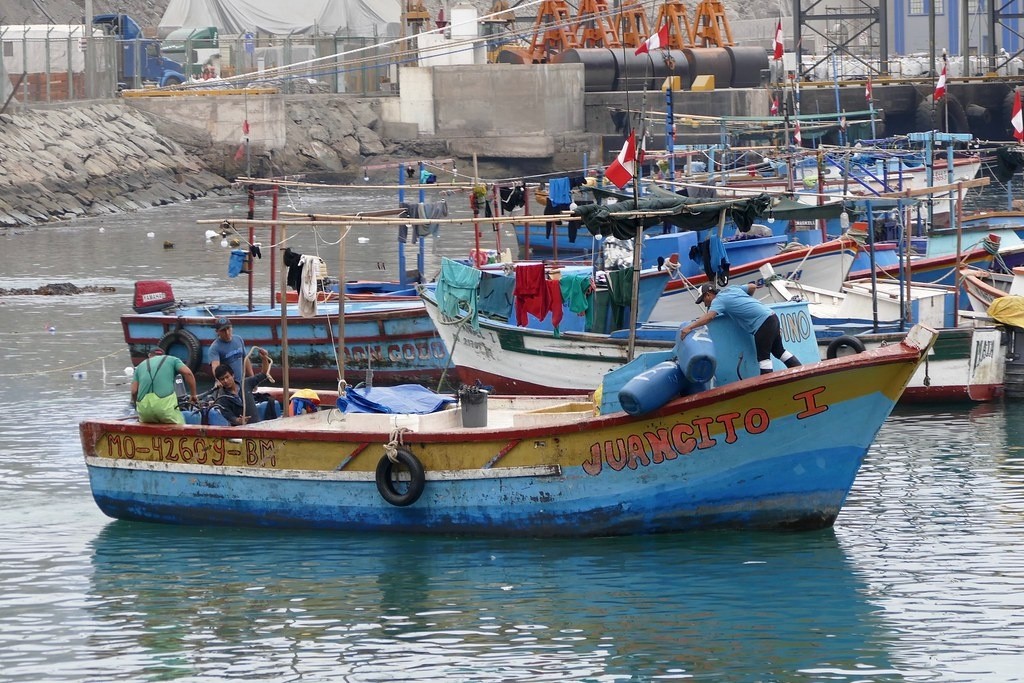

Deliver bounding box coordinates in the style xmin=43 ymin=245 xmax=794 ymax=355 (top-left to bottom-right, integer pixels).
xmin=83 ymin=15 xmax=1023 ymax=532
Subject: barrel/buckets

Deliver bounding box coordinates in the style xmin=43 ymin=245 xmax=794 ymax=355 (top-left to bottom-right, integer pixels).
xmin=208 ymin=408 xmax=228 ymax=426
xmin=460 ymin=392 xmax=488 ymax=427
xmin=674 ymin=319 xmax=716 ymax=384
xmin=619 ymin=358 xmax=685 ymax=415
xmin=181 ymin=408 xmax=201 ymax=425
xmin=255 ymin=400 xmax=280 ymax=421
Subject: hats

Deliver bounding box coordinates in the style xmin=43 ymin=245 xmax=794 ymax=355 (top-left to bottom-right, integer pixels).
xmin=214 ymin=317 xmax=232 ymax=331
xmin=149 ymin=347 xmax=165 ymax=355
xmin=694 ymin=283 xmax=716 ymax=304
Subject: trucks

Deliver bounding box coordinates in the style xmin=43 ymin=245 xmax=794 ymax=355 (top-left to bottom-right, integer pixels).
xmin=160 ymin=25 xmax=218 ymax=76
xmin=90 ymin=15 xmax=186 ymax=87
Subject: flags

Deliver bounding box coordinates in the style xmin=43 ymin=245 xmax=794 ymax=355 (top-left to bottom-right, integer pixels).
xmin=774 ymin=23 xmax=786 ymax=63
xmin=603 ymin=128 xmax=638 ymax=191
xmin=1011 ymin=89 xmax=1023 ymax=143
xmin=635 ymin=23 xmax=671 ymax=56
xmin=770 ymin=99 xmax=779 ymax=113
xmin=792 ymin=120 xmax=802 ymax=144
xmin=864 ymin=79 xmax=873 ymax=102
xmin=933 ymin=64 xmax=947 ymax=100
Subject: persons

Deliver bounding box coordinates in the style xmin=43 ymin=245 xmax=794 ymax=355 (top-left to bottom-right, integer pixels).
xmin=131 ymin=346 xmax=199 ymax=426
xmin=214 ymin=349 xmax=271 ymax=427
xmin=681 ymin=282 xmax=803 ymax=376
xmin=207 ymin=316 xmax=258 ymax=394
